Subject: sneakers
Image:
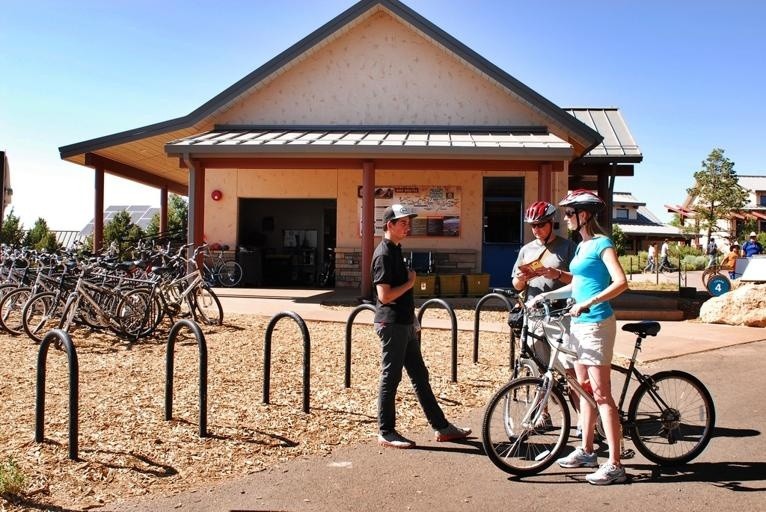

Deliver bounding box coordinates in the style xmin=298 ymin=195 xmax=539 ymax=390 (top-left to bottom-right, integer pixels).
xmin=585 ymin=462 xmax=627 ymax=485
xmin=435 ymin=423 xmax=472 ymax=442
xmin=377 ymin=431 xmax=416 ymax=448
xmin=558 ymin=447 xmax=598 ymax=468
xmin=534 ymin=413 xmax=553 ymax=430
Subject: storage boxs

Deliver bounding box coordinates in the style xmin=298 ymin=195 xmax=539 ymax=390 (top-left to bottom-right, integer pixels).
xmin=438 ymin=273 xmax=463 ymax=295
xmin=412 ymin=273 xmax=437 ymax=295
xmin=465 ymin=272 xmax=491 ymax=294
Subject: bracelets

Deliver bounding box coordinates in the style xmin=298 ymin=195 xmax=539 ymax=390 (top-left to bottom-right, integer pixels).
xmin=554 ymin=268 xmax=563 ymax=282
xmin=589 ymin=296 xmax=600 ymax=305
xmin=539 ymin=294 xmax=546 ymax=299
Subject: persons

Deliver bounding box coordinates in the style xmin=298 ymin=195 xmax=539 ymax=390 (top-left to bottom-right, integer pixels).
xmin=521 ymin=186 xmax=630 ymax=486
xmin=706 ymin=235 xmax=718 ymax=274
xmin=642 ymin=240 xmax=657 ymax=274
xmin=719 ymin=242 xmax=741 ymax=280
xmin=369 ymin=203 xmax=473 ymax=450
xmin=658 ymin=237 xmax=673 ymax=274
xmin=741 ymin=231 xmax=764 ymax=257
xmin=509 ymin=200 xmax=580 ymax=434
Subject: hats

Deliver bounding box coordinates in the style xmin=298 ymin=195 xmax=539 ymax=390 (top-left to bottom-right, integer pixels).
xmin=749 ymin=231 xmax=756 ymax=236
xmin=730 ymin=241 xmax=740 ymax=251
xmin=383 ymin=204 xmax=418 ymax=223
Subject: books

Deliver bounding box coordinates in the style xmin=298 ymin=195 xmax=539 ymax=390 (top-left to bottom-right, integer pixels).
xmin=517 ymin=259 xmax=545 ymax=280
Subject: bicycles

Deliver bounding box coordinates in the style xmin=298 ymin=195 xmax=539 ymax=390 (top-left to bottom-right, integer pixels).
xmin=492 ymin=287 xmax=576 ymax=443
xmin=0 ymin=230 xmax=243 ymax=346
xmin=317 ymin=246 xmax=335 ymax=288
xmin=480 ymin=301 xmax=716 ymax=477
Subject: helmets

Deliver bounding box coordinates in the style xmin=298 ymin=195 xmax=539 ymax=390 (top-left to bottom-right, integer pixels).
xmin=558 ymin=188 xmax=606 ymax=207
xmin=524 ymin=201 xmax=556 ymax=225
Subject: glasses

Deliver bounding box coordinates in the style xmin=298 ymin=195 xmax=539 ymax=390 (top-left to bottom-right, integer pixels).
xmin=565 ymin=209 xmax=583 ymax=218
xmin=532 ymin=218 xmax=552 ymax=228
